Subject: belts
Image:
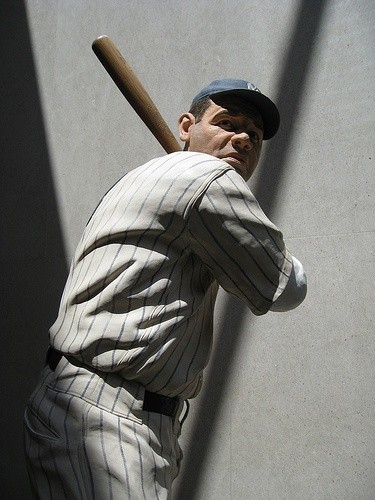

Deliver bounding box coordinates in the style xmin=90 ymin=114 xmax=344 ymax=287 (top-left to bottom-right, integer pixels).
xmin=48 ymin=350 xmax=180 ymax=417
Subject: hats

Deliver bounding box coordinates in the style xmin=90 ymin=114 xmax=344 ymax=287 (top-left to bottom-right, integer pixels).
xmin=192 ymin=78 xmax=283 ymax=140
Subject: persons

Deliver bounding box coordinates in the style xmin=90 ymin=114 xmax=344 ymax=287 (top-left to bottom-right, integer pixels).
xmin=20 ymin=77 xmax=307 ymax=500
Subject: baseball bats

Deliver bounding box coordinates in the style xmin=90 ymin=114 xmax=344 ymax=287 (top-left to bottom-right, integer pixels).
xmin=91 ymin=34 xmax=182 ymax=154
